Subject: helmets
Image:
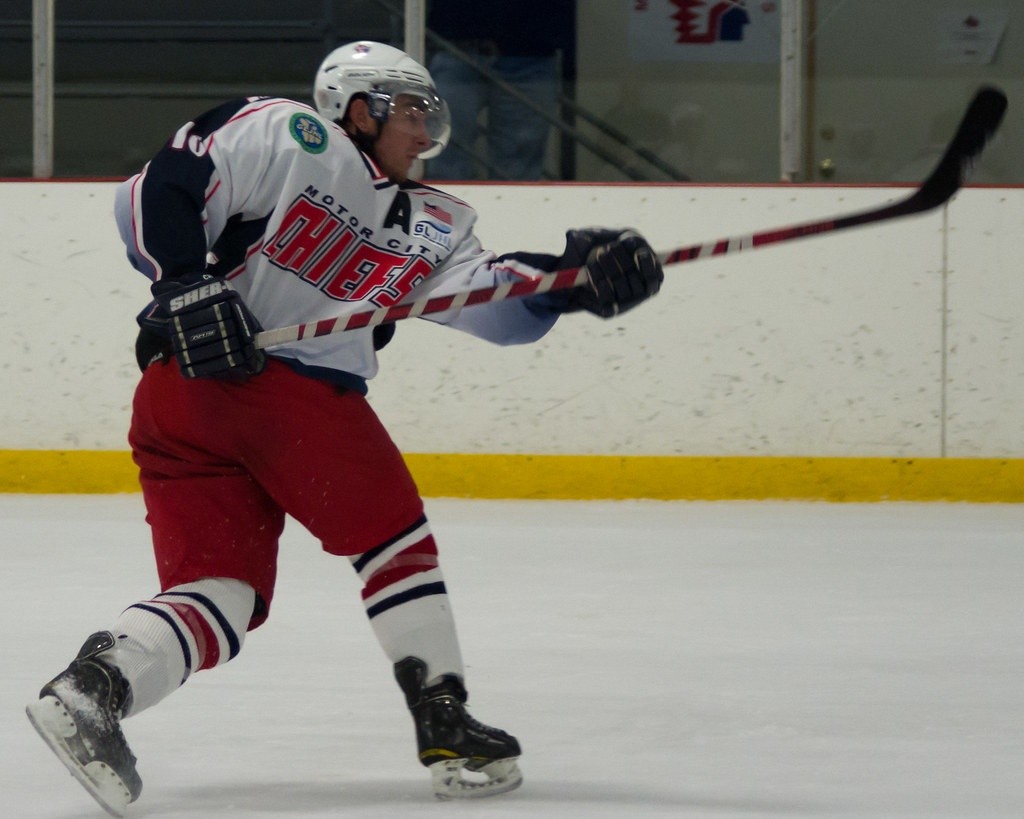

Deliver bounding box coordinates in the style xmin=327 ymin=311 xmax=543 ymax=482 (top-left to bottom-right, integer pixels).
xmin=311 ymin=38 xmax=440 ymax=124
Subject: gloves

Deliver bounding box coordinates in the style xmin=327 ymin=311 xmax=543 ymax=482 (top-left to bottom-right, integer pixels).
xmin=151 ymin=276 xmax=269 ymax=383
xmin=553 ymin=224 xmax=666 ymax=320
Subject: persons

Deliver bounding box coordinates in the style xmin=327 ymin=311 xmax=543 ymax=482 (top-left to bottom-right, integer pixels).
xmin=24 ymin=41 xmax=666 ymax=819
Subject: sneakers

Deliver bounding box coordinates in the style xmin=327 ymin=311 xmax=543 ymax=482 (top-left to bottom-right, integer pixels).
xmin=394 ymin=655 xmax=523 ymax=802
xmin=27 ymin=627 xmax=145 ymax=819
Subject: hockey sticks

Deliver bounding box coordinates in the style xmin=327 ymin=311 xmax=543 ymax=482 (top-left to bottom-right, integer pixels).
xmin=252 ymin=83 xmax=1009 ymax=350
